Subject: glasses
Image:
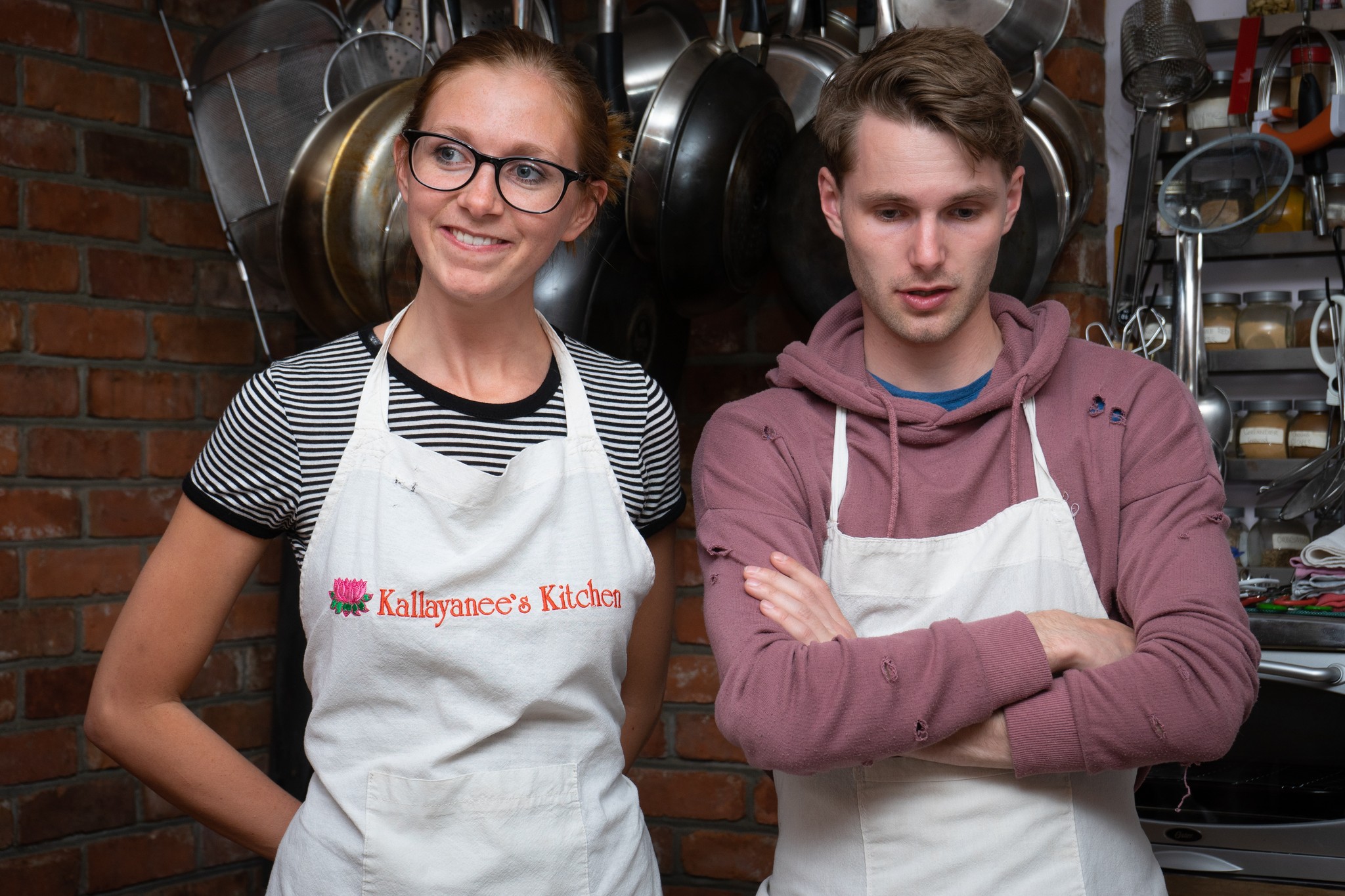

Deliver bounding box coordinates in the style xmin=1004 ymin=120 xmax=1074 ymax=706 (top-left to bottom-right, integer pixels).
xmin=403 ymin=128 xmax=591 ymax=212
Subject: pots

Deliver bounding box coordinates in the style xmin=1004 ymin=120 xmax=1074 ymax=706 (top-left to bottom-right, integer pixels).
xmin=158 ymin=1 xmax=1099 ymax=401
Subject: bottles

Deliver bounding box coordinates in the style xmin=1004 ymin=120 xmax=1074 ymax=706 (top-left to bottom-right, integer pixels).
xmin=1287 ymin=399 xmax=1338 ymax=459
xmin=1143 ymin=288 xmax=1342 ymax=350
xmin=1223 ymin=400 xmax=1243 ymax=460
xmin=1151 ymin=174 xmax=1344 ymax=238
xmin=1156 ymin=42 xmax=1342 ymax=132
xmin=1223 ymin=506 xmax=1249 ymax=566
xmin=1241 ymin=402 xmax=1291 ymax=460
xmin=1310 ymin=504 xmax=1344 ymax=542
xmin=1248 ymin=507 xmax=1312 ymax=568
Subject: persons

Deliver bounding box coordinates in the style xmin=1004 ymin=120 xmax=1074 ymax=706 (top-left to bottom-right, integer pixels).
xmin=689 ymin=17 xmax=1255 ymax=896
xmin=75 ymin=25 xmax=689 ymax=896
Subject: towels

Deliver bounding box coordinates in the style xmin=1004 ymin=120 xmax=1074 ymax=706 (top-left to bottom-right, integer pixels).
xmin=1289 ymin=523 xmax=1345 ymax=609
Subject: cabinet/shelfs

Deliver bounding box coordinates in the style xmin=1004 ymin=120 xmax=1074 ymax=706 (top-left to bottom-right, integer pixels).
xmin=1128 ymin=3 xmax=1345 ymax=594
